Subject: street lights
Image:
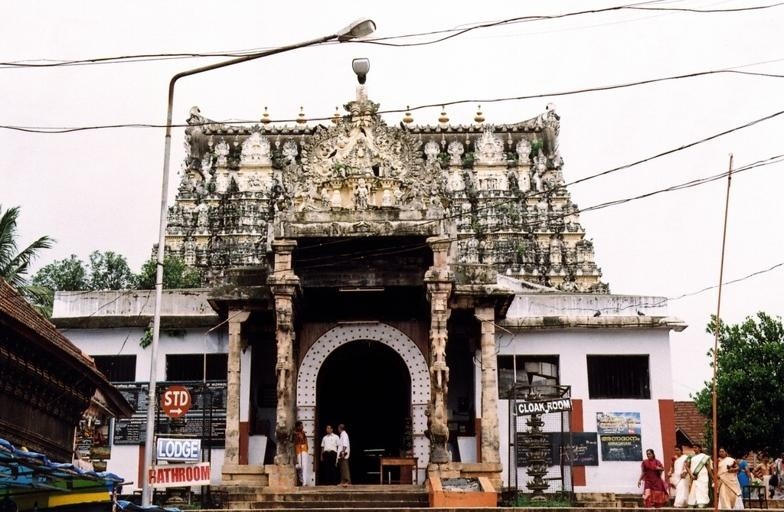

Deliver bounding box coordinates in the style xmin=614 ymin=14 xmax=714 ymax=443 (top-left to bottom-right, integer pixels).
xmin=142 ymin=16 xmax=375 ymax=506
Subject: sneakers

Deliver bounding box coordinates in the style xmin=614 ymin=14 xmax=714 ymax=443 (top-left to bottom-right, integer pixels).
xmin=337 ymin=482 xmax=351 ymax=487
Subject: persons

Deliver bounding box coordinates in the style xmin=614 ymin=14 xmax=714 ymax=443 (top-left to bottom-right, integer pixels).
xmin=637 ymin=449 xmax=669 ymax=508
xmin=320 ymin=424 xmax=339 ymax=485
xmin=666 ymin=443 xmax=784 ymax=510
xmin=335 ymin=424 xmax=352 ymax=487
xmin=293 ymin=421 xmax=309 ymax=487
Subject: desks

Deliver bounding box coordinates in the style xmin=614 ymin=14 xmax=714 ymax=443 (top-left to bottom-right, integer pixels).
xmin=380 ymin=457 xmax=419 ymax=485
xmin=743 ymin=485 xmax=768 ymax=509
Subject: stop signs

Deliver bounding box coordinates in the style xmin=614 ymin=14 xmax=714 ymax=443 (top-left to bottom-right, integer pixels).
xmin=160 ymin=385 xmax=193 ymax=417
